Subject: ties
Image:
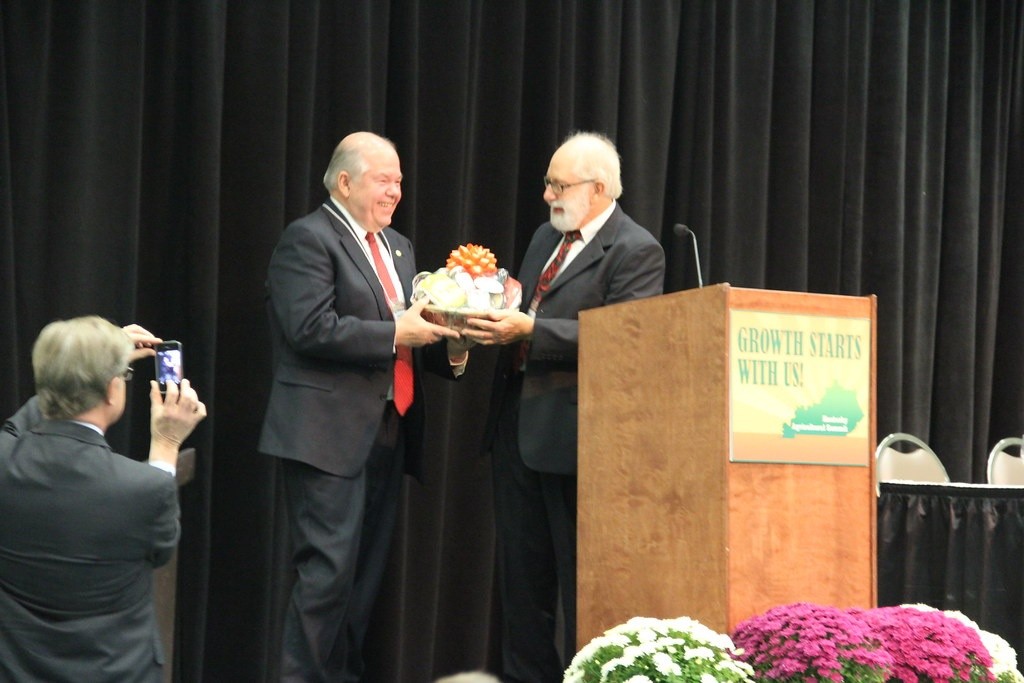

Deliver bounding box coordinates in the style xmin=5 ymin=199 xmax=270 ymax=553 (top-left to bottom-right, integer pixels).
xmin=364 ymin=231 xmax=413 ymax=416
xmin=513 ymin=229 xmax=581 ymax=368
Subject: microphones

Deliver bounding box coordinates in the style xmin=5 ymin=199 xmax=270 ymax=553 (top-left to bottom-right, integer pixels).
xmin=673 ymin=223 xmax=703 ymax=289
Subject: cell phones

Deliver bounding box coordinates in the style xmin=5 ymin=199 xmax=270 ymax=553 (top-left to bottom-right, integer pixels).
xmin=154 ymin=341 xmax=184 ymax=396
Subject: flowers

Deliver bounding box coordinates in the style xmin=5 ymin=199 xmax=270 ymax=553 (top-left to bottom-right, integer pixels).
xmin=903 ymin=601 xmax=1024 ymax=683
xmin=558 ymin=615 xmax=757 ymax=683
xmin=730 ymin=604 xmax=896 ymax=683
xmin=854 ymin=603 xmax=998 ymax=683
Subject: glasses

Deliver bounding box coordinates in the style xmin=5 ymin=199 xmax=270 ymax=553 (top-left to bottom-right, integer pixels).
xmin=120 ymin=367 xmax=135 ymax=381
xmin=543 ymin=176 xmax=596 ymax=194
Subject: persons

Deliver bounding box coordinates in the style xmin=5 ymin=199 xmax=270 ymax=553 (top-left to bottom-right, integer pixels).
xmin=254 ymin=129 xmax=482 ymax=683
xmin=456 ymin=132 xmax=673 ymax=683
xmin=0 ymin=315 xmax=206 ymax=682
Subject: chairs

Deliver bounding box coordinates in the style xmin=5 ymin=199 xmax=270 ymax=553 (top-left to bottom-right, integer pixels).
xmin=985 ymin=437 xmax=1024 ymax=488
xmin=875 ymin=433 xmax=950 ymax=497
